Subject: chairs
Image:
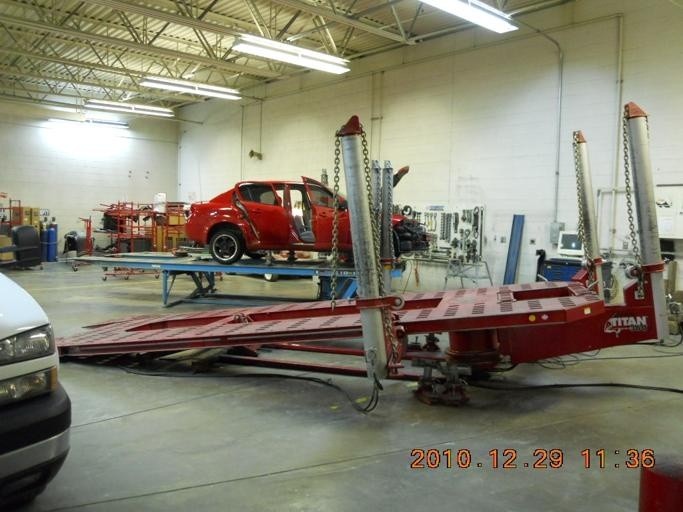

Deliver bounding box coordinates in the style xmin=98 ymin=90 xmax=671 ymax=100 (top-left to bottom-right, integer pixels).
xmin=258 ymin=191 xmax=317 ymax=243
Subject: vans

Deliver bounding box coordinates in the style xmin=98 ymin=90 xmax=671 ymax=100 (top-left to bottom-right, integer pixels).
xmin=0 ymin=273 xmax=72 ymax=507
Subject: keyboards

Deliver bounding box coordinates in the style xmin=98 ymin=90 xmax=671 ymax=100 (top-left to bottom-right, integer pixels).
xmin=550 ymin=257 xmax=583 ymax=262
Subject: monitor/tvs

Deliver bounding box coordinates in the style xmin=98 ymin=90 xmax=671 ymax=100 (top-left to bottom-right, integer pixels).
xmin=557 ymin=231 xmax=584 ymax=258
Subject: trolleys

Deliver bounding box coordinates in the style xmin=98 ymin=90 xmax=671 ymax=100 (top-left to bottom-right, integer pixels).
xmin=71 ymin=201 xmax=184 ymax=281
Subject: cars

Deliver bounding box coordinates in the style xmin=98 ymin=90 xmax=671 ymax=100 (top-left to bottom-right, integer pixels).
xmin=185 ymin=177 xmax=429 ymax=265
